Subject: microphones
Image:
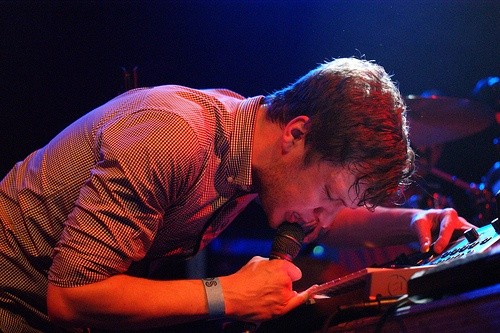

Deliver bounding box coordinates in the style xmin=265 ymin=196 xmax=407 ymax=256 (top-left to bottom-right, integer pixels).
xmin=431 ymin=167 xmax=470 ymax=189
xmin=241 ymin=224 xmax=305 ymax=333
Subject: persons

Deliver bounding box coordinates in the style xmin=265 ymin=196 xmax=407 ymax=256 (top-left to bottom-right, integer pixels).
xmin=0 ymin=56 xmax=481 ymax=332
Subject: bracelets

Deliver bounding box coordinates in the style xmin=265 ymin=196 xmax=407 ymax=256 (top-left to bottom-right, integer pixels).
xmin=201 ymin=276 xmax=226 ymax=318
